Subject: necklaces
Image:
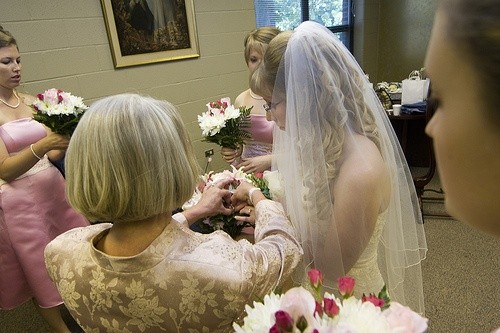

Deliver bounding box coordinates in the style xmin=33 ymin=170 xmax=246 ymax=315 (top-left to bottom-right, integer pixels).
xmin=0 ymin=92 xmax=20 ymax=108
xmin=250 ymin=92 xmax=263 ymax=100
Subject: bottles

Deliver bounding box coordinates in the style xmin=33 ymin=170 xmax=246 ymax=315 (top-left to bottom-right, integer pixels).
xmin=408 ymin=70 xmax=423 ymax=80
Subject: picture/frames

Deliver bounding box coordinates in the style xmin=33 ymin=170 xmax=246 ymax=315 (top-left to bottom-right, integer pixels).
xmin=100 ymin=0 xmax=201 ymax=70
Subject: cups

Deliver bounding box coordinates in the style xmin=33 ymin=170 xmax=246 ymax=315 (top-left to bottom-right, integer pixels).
xmin=387 ymin=109 xmax=393 ymax=116
xmin=392 ymin=104 xmax=402 ymax=116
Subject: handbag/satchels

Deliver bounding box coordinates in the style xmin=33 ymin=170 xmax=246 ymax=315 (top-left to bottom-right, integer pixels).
xmin=401 ymin=70 xmax=430 ymax=108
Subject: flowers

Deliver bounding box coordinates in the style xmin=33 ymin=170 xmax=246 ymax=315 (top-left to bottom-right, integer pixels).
xmin=181 ymin=164 xmax=273 ymax=240
xmin=232 ymin=269 xmax=429 ymax=333
xmin=197 ymin=96 xmax=254 ymax=151
xmin=25 ymin=88 xmax=89 ymax=137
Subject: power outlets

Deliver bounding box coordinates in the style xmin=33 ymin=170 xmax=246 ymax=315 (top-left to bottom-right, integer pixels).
xmin=204 ymin=148 xmax=214 ymax=158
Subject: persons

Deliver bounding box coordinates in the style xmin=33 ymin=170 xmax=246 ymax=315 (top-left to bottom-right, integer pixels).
xmin=221 ymin=27 xmax=282 ymax=178
xmin=44 ymin=94 xmax=303 ymax=333
xmin=0 ymin=25 xmax=90 ymax=333
xmin=425 ymin=0 xmax=500 ymax=236
xmin=250 ymin=20 xmax=428 ymax=315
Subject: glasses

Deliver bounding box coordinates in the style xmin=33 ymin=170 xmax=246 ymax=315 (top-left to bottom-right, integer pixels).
xmin=262 ymin=98 xmax=285 ymax=112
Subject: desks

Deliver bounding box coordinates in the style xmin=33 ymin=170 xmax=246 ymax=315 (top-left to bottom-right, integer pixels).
xmin=388 ymin=113 xmax=427 ymax=161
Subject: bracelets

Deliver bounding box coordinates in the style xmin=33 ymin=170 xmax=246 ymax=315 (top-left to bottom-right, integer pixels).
xmin=31 ymin=144 xmax=44 ymax=160
xmin=249 ymin=188 xmax=261 ymax=204
